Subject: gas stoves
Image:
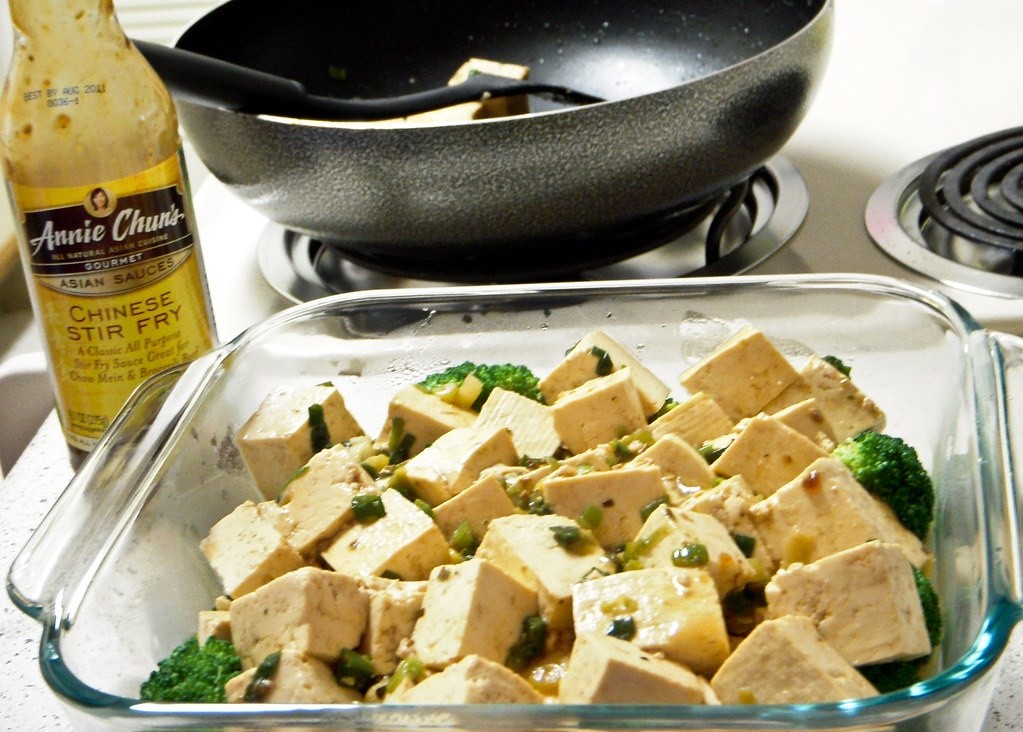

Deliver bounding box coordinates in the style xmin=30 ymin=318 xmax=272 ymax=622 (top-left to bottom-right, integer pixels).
xmin=256 ymin=123 xmax=1023 ymax=321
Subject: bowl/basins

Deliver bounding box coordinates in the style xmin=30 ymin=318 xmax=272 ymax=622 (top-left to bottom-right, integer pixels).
xmin=6 ymin=269 xmax=1023 ymax=732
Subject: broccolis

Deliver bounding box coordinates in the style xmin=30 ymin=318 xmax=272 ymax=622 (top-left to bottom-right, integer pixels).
xmin=139 ymin=359 xmax=944 ymax=711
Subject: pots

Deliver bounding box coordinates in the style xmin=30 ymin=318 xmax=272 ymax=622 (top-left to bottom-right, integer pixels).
xmin=173 ymin=0 xmax=835 ymax=246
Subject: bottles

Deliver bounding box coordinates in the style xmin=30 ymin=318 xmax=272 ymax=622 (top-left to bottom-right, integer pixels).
xmin=2 ymin=0 xmax=221 ymax=480
